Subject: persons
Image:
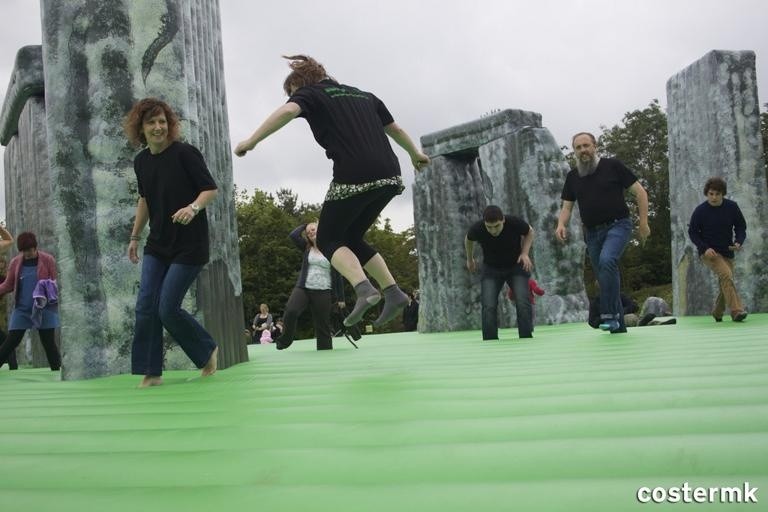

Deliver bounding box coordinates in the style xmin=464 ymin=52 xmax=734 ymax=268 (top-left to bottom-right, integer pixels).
xmin=0 ymin=232 xmax=62 ymax=371
xmin=403 ymin=294 xmax=419 ymax=332
xmin=0 ymin=221 xmax=18 ymax=370
xmin=555 ymin=132 xmax=651 ymax=334
xmin=275 ymin=222 xmax=346 ymax=351
xmin=252 ymin=303 xmax=273 ymax=344
xmin=235 ymin=54 xmax=430 ymax=328
xmin=588 ymin=279 xmax=676 ymax=329
xmin=464 ymin=205 xmax=535 ymax=340
xmin=508 ymin=278 xmax=545 ymax=332
xmin=688 ymin=177 xmax=748 ymax=322
xmin=123 ymin=98 xmax=219 ymax=388
xmin=271 ymin=317 xmax=284 ymax=343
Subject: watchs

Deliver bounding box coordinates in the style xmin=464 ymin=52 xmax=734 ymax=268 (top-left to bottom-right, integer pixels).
xmin=189 ymin=204 xmax=199 ymax=216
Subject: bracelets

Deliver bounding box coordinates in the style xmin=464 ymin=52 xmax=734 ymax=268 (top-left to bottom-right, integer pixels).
xmin=129 ymin=235 xmax=141 ymax=241
